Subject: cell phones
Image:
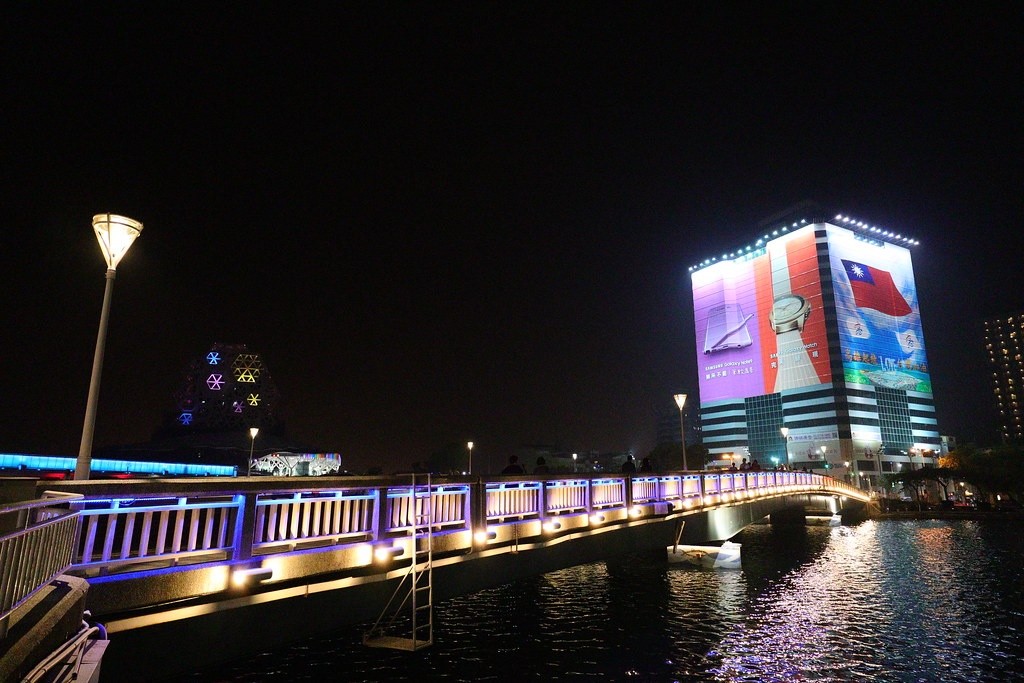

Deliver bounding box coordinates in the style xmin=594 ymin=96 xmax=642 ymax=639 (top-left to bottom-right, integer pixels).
xmin=704 ymin=302 xmax=752 ymax=355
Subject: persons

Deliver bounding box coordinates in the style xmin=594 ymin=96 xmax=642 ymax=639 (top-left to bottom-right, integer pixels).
xmin=620 ymin=455 xmax=636 ymax=474
xmin=768 ymin=246 xmax=821 ymax=393
xmin=748 ymin=462 xmax=752 ymax=469
xmin=641 ymin=457 xmax=653 ymax=473
xmin=773 ymin=464 xmax=813 ymax=473
xmin=531 ymin=456 xmax=553 ymax=475
xmin=500 ymin=454 xmax=523 ymax=475
xmin=739 ymin=458 xmax=750 ymax=471
xmin=752 ymin=460 xmax=760 ymax=469
xmin=728 ymin=463 xmax=737 ymax=471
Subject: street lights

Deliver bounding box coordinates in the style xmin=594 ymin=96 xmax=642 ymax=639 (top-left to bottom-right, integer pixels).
xmin=247 ymin=427 xmax=260 ymax=476
xmin=780 ymin=427 xmax=789 ymax=470
xmin=674 ymin=394 xmax=688 ymax=470
xmin=910 ymin=448 xmax=931 ymax=502
xmin=844 ymin=462 xmax=850 ymax=484
xmin=467 ymin=441 xmax=474 ymax=474
xmin=821 ymin=445 xmax=827 ymax=475
xmin=72 ymin=211 xmax=143 ymax=479
